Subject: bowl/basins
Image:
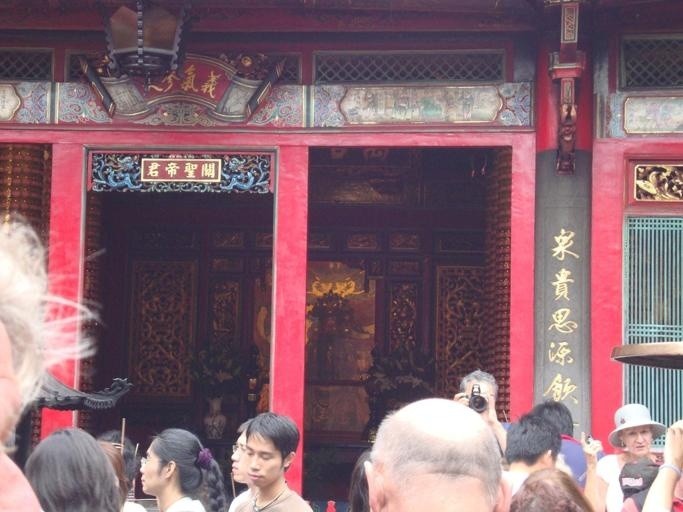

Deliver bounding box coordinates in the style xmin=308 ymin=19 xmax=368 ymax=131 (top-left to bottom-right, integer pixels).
xmin=253 ymin=488 xmax=285 ymax=512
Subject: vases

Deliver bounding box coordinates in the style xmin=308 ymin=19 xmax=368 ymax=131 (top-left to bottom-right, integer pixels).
xmin=188 ymin=340 xmax=242 ymax=401
xmin=371 ymin=349 xmax=433 ymax=393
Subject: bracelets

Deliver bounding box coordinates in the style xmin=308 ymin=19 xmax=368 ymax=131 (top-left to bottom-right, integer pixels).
xmin=658 ymin=463 xmax=681 ymax=482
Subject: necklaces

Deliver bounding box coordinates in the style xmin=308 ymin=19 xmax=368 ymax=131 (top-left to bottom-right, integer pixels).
xmin=253 ymin=488 xmax=285 ymax=512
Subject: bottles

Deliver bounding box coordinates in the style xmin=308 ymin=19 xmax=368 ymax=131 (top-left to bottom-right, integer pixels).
xmin=619 ymin=459 xmax=657 ymax=502
xmin=605 ymin=403 xmax=667 ymax=448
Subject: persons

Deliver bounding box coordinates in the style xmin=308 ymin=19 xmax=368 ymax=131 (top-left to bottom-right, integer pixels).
xmin=224 ymin=416 xmax=253 ymax=512
xmin=234 ymin=413 xmax=315 ymax=512
xmin=22 ymin=425 xmax=122 ymax=512
xmin=97 ymin=430 xmax=146 ymax=511
xmin=138 ymin=428 xmax=227 ymax=511
xmin=95 ymin=438 xmax=130 ymax=512
xmin=348 ymin=369 xmax=683 ymax=512
xmin=0 ymin=212 xmax=93 ymax=512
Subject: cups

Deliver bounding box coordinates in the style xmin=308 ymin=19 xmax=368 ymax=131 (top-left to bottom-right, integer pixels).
xmin=232 ymin=444 xmax=242 ymax=453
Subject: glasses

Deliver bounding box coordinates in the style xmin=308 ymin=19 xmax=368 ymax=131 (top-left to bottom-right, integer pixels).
xmin=98 ymin=0 xmax=191 ymax=93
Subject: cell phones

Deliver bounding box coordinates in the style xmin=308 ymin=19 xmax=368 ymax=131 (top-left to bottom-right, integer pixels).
xmin=460 ymin=384 xmax=487 ymax=414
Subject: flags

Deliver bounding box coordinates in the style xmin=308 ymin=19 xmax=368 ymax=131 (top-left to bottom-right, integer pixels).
xmin=203 ymin=396 xmax=226 ymax=441
